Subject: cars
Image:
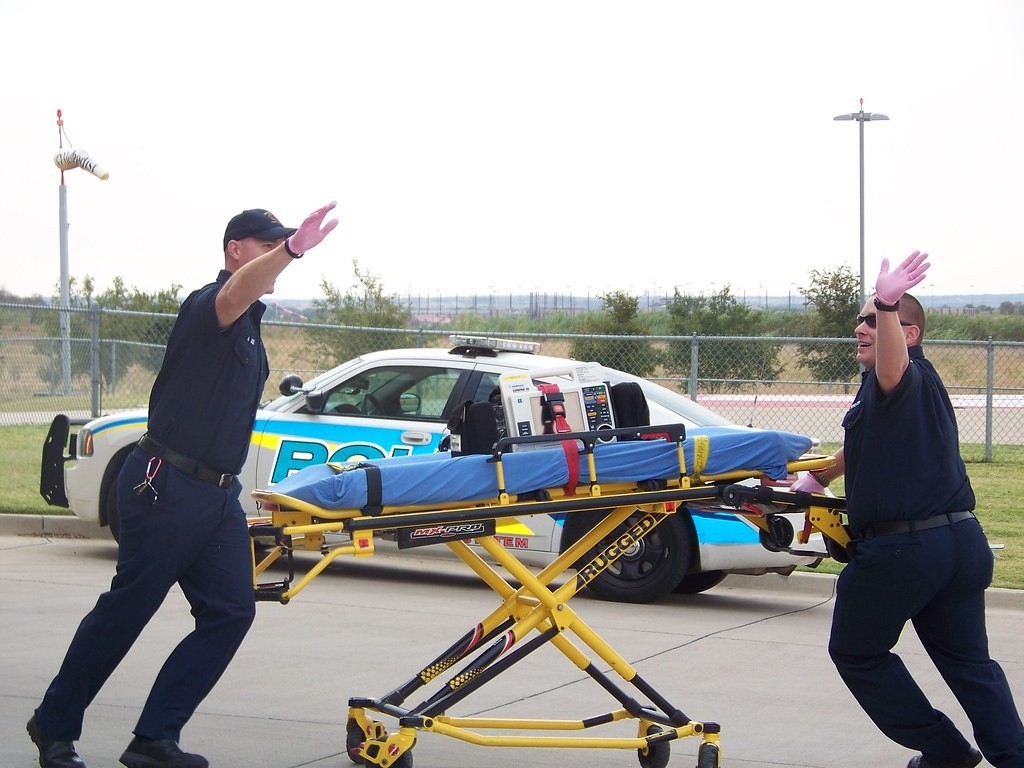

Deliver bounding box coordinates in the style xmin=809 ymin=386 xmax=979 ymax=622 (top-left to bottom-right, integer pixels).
xmin=37 ymin=336 xmax=840 ymax=603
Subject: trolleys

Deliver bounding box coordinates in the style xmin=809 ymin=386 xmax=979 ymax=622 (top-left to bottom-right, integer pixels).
xmin=244 ymin=366 xmax=858 ymax=768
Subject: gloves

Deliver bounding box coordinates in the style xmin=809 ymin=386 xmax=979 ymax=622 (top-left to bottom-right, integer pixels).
xmin=289 ymin=201 xmax=339 ymax=255
xmin=789 ymin=472 xmax=825 ymax=495
xmin=875 ymin=250 xmax=931 ymax=306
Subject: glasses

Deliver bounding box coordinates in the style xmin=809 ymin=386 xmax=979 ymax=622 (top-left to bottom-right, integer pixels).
xmin=856 ymin=314 xmax=913 ymax=328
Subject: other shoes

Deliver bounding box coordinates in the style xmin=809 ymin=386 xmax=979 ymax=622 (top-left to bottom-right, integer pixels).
xmin=907 ymin=746 xmax=983 ymax=768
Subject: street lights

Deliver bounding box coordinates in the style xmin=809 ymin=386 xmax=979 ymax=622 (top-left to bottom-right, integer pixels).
xmin=834 ymin=113 xmax=890 ymax=312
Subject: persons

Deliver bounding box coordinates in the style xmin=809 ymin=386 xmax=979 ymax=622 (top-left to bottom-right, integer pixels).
xmin=28 ymin=201 xmax=338 ymax=768
xmin=791 ymin=250 xmax=1024 ymax=767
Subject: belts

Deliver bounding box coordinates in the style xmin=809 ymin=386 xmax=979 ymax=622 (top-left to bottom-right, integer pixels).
xmin=864 ymin=509 xmax=976 ymax=540
xmin=138 ymin=436 xmax=233 ymax=489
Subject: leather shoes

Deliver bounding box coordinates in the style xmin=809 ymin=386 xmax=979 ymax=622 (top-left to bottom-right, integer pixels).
xmin=119 ymin=735 xmax=209 ymax=768
xmin=26 ymin=715 xmax=86 ymax=768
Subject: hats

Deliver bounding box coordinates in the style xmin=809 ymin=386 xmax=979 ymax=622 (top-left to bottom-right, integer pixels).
xmin=223 ymin=208 xmax=298 ymax=250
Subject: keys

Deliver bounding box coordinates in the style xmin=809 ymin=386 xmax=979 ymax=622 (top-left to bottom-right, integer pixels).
xmin=133 ymin=481 xmax=159 ymax=496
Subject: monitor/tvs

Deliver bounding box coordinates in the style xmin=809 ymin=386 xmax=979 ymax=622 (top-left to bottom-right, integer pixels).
xmin=530 ymin=391 xmax=585 ymax=435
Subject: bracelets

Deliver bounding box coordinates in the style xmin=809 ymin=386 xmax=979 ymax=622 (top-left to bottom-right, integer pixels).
xmin=872 ymin=298 xmax=900 ymax=312
xmin=809 ymin=471 xmax=829 ymax=487
xmin=285 ymin=237 xmax=303 ymax=259
